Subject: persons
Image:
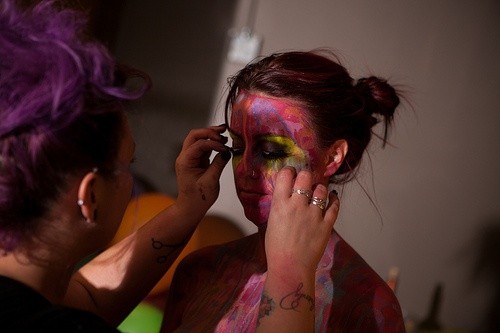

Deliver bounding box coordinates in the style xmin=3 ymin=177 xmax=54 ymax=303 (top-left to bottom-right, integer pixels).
xmin=160 ymin=48 xmax=405 ymax=333
xmin=0 ymin=0 xmax=343 ymax=333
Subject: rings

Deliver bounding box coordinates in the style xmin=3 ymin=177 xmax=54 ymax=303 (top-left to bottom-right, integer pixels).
xmin=289 ymin=186 xmax=312 ymax=200
xmin=310 ymin=195 xmax=328 ymax=210
